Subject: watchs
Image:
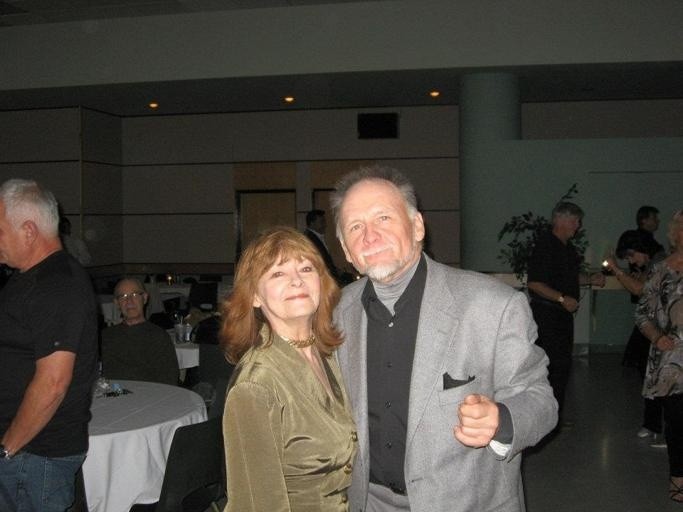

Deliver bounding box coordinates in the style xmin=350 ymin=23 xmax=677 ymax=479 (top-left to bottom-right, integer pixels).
xmin=557 ymin=294 xmax=568 ymax=306
xmin=0 ymin=443 xmax=10 ymax=460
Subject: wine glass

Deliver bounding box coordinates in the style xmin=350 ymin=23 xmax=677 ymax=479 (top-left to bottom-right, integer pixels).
xmin=149 ymin=270 xmax=156 ymax=284
xmin=166 ymin=272 xmax=173 ymax=286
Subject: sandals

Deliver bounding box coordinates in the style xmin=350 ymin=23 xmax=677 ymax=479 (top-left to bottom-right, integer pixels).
xmin=666 ymin=473 xmax=683 ymax=506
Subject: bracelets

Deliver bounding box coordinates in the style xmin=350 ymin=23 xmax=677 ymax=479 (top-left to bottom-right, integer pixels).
xmin=655 ymin=334 xmax=663 ymax=345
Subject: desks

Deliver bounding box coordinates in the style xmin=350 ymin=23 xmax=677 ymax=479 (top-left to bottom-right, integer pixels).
xmin=486 ymin=272 xmax=627 ymax=344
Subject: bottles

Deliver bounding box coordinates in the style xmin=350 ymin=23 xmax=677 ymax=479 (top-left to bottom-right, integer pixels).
xmin=185 ymin=323 xmax=193 ymax=341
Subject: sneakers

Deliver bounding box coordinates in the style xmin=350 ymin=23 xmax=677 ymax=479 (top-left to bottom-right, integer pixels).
xmin=635 ymin=422 xmax=670 ymax=448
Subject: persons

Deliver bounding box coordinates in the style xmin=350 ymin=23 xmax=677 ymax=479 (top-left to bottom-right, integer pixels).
xmin=600 ymin=230 xmax=668 ymax=448
xmin=329 ymin=162 xmax=560 ymax=512
xmin=99 ymin=278 xmax=180 ymax=387
xmin=300 ymin=209 xmax=337 ymax=280
xmin=629 ymin=203 xmax=683 ymax=504
xmin=217 ymin=225 xmax=360 ymax=511
xmin=617 ymin=205 xmax=664 ymax=371
xmin=1 ymin=177 xmax=98 ymax=512
xmin=527 ymin=199 xmax=605 ymax=413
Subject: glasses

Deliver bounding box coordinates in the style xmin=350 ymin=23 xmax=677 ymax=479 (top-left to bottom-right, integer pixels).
xmin=116 ymin=290 xmax=144 ymax=301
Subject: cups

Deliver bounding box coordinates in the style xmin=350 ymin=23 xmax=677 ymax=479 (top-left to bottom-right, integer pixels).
xmin=175 ymin=325 xmax=185 ymax=344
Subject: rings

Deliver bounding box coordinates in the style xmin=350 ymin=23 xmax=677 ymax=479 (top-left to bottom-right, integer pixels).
xmin=664 ymin=341 xmax=668 ymax=345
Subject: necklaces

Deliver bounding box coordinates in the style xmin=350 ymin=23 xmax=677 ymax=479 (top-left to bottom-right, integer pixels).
xmin=279 ymin=334 xmax=319 ymax=348
